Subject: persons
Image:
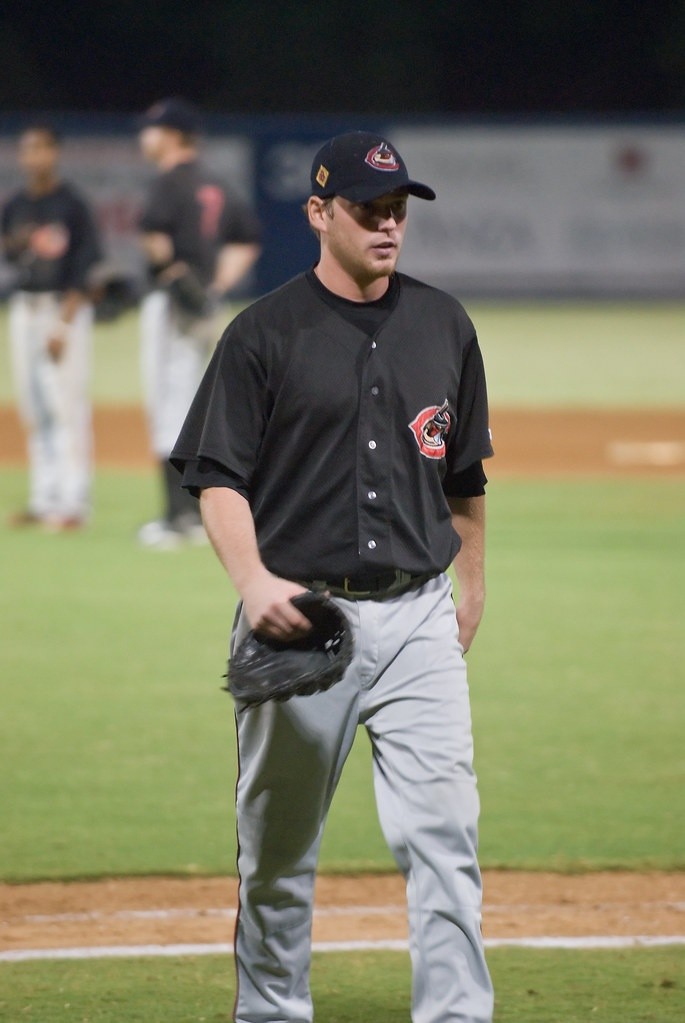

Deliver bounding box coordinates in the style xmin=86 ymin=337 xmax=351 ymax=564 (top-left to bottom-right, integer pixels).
xmin=169 ymin=130 xmax=493 ymax=1023
xmin=136 ymin=100 xmax=263 ymax=549
xmin=0 ymin=125 xmax=105 ymax=529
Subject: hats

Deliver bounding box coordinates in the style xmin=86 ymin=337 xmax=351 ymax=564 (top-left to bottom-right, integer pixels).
xmin=134 ymin=97 xmax=201 ymax=132
xmin=309 ymin=132 xmax=436 ymax=205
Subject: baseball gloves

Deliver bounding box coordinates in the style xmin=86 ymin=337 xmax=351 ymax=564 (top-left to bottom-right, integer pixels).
xmin=226 ymin=591 xmax=354 ymax=704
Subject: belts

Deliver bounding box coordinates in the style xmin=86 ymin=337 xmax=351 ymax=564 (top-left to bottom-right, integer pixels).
xmin=285 ymin=569 xmax=447 ymax=601
